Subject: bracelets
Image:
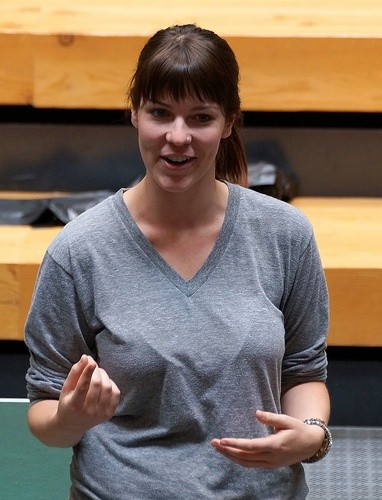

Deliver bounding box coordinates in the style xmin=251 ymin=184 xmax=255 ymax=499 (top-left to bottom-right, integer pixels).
xmin=300 ymin=417 xmax=332 ymax=463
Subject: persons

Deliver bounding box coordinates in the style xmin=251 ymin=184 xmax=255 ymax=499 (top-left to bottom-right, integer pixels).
xmin=22 ymin=17 xmax=335 ymax=500
